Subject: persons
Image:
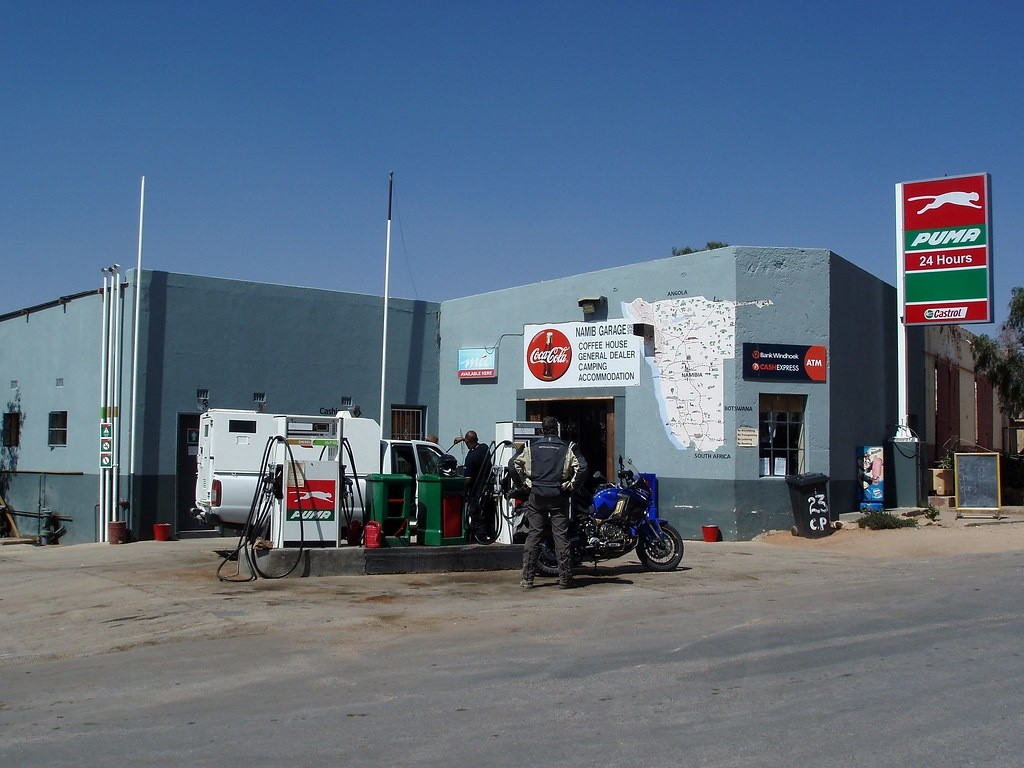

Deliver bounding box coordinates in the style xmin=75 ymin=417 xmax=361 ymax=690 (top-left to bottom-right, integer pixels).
xmin=508 ymin=417 xmax=588 ymax=589
xmin=404 ymin=434 xmax=438 ymax=471
xmin=454 ymin=431 xmax=496 ymax=542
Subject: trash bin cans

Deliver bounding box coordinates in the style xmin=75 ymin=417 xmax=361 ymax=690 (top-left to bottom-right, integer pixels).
xmin=786 ymin=471 xmax=834 ymax=539
xmin=365 ymin=473 xmax=412 ymax=547
xmin=417 ymin=474 xmax=471 ymax=546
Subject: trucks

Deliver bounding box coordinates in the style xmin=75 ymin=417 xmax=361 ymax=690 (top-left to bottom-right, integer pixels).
xmin=191 ymin=408 xmax=465 ymax=537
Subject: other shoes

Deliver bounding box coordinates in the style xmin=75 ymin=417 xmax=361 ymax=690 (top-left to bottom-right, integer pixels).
xmin=520 ymin=579 xmax=533 ymax=588
xmin=559 ymin=578 xmax=578 ymax=588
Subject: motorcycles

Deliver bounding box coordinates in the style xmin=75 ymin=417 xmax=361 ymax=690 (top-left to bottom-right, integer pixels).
xmin=506 ymin=454 xmax=685 ymax=577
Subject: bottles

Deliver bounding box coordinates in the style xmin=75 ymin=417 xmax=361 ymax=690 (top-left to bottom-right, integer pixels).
xmin=542 ymin=333 xmax=553 ymax=378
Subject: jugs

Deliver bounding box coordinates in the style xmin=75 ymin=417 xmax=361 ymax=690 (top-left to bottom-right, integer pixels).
xmin=347 ymin=520 xmax=362 ymax=545
xmin=364 ymin=521 xmax=382 ymax=548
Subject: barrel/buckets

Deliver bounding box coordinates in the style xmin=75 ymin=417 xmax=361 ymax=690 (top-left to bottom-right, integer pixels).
xmin=702 ymin=524 xmax=719 ymax=541
xmin=108 ymin=521 xmax=126 ymax=544
xmin=153 ymin=523 xmax=171 ymax=541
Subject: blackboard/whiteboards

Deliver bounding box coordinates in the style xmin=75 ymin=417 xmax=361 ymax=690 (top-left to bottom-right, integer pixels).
xmin=954 ymin=453 xmax=1001 ymax=511
